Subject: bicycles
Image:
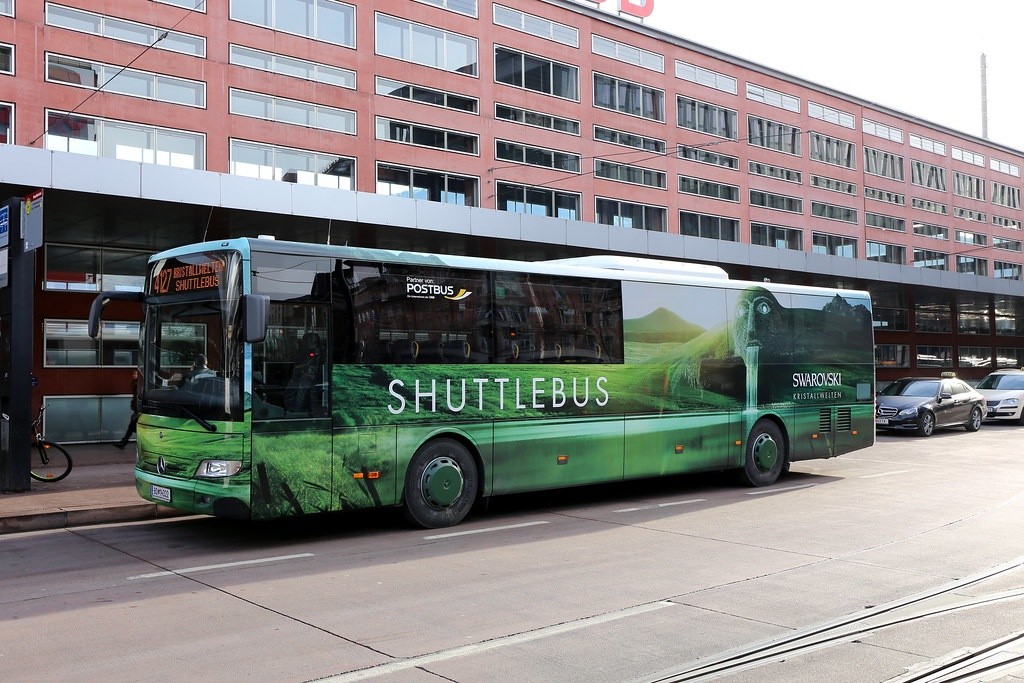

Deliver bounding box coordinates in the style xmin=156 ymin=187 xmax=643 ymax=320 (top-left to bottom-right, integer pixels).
xmin=1 ymin=404 xmax=73 ymax=485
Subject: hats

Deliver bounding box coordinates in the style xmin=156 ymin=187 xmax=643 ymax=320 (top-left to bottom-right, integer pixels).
xmin=196 ymin=354 xmax=208 ymax=366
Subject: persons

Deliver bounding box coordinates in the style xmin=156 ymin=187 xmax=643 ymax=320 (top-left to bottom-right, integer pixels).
xmin=181 ymin=354 xmax=218 ymax=383
xmin=112 ymin=370 xmax=138 ymax=449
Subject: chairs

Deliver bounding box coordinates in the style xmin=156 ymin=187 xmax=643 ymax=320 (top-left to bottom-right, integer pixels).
xmin=388 ymin=339 xmax=419 ymax=365
xmin=439 ymin=338 xmax=602 ymax=364
xmin=283 ymin=333 xmax=322 ymax=411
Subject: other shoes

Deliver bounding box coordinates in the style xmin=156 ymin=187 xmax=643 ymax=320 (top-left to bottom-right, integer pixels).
xmin=112 ymin=441 xmax=125 ymax=449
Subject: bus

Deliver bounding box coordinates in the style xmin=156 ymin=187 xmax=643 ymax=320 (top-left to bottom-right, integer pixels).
xmin=87 ymin=233 xmax=877 ymax=531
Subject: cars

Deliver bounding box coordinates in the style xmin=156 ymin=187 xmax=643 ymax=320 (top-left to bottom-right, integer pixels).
xmin=973 ymin=367 xmax=1024 ymax=426
xmin=874 ymin=370 xmax=988 ymax=437
